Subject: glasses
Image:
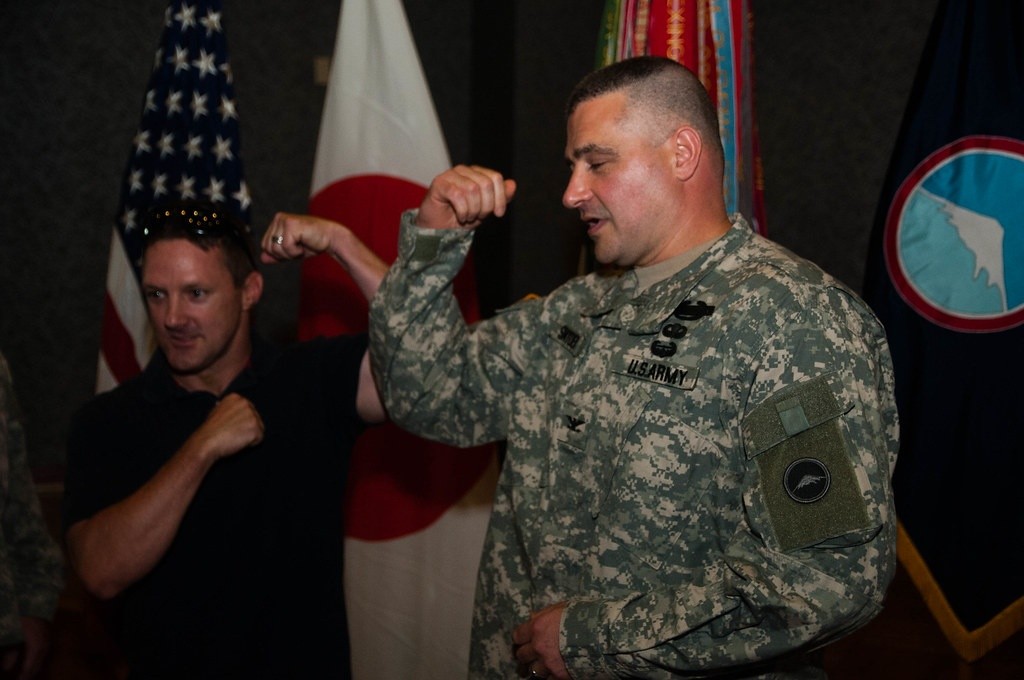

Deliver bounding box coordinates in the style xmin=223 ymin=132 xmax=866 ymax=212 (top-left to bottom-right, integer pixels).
xmin=139 ymin=209 xmax=249 ymax=263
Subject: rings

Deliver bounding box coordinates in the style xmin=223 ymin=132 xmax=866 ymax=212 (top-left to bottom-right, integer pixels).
xmin=271 ymin=235 xmax=283 ymax=244
xmin=529 ymin=665 xmax=546 ymax=678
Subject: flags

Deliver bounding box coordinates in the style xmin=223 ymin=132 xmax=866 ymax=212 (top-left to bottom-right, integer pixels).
xmin=92 ymin=0 xmax=249 ymax=393
xmin=599 ymin=0 xmax=770 ymax=237
xmin=306 ymin=0 xmax=503 ymax=680
xmin=860 ymin=0 xmax=1024 ymax=659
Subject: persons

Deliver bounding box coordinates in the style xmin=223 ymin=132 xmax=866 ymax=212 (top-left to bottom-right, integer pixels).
xmin=368 ymin=54 xmax=900 ymax=680
xmin=0 ymin=349 xmax=69 ymax=680
xmin=62 ymin=199 xmax=388 ymax=680
